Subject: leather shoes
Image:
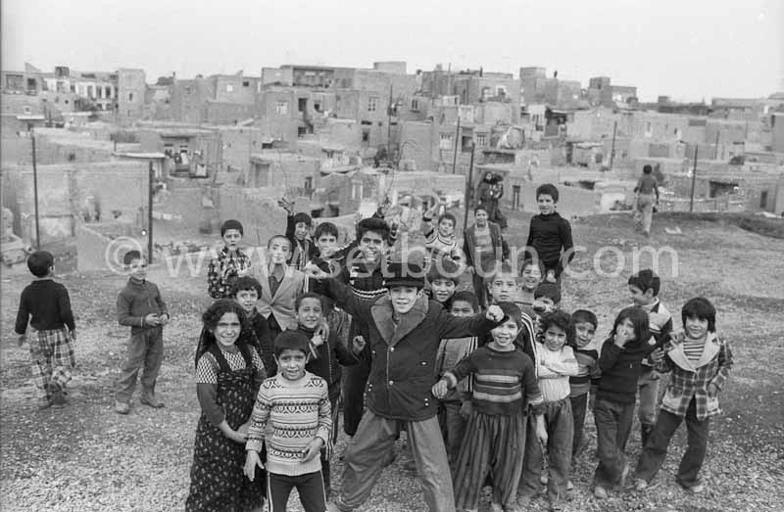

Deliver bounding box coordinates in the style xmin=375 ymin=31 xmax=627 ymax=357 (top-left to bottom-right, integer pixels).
xmin=114 ymin=399 xmax=130 ymax=414
xmin=37 ymin=399 xmax=55 ymax=409
xmin=141 ymin=391 xmax=164 ymax=408
xmin=51 ymin=383 xmax=68 ymax=405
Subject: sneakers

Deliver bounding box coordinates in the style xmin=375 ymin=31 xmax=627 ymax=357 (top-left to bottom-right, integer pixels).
xmin=592 ymin=485 xmax=607 ymax=499
xmin=680 ymin=477 xmax=703 ymax=492
xmin=618 ymin=462 xmax=632 ymax=488
xmin=633 ymin=475 xmax=649 ymax=491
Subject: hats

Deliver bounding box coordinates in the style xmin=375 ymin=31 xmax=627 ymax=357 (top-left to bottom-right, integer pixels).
xmin=384 ymin=264 xmax=425 ymax=290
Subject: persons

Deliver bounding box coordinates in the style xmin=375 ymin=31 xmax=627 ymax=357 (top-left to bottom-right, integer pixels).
xmin=305 ymin=259 xmax=511 ymax=512
xmin=338 ymin=217 xmax=398 ymax=468
xmin=461 ymin=263 xmax=540 ymax=417
xmin=431 ymin=303 xmax=546 ymax=512
xmin=278 ymin=197 xmax=313 ymax=267
xmin=295 ymin=295 xmax=367 ymax=502
xmin=185 ymin=301 xmax=267 ymax=511
xmin=533 ymin=312 xmax=580 ymax=491
xmin=629 ymin=270 xmax=673 ymax=445
xmin=524 ymin=185 xmax=575 ymax=284
xmin=513 ymin=259 xmax=543 ymax=316
xmin=15 ymin=252 xmax=76 ymax=408
xmin=208 ymin=221 xmax=250 ymax=301
xmin=572 ymin=310 xmax=600 ymax=458
xmin=427 ymin=213 xmax=458 ymax=263
xmin=233 ymin=278 xmax=276 ymax=377
xmin=533 ymin=284 xmax=561 ymax=316
xmin=426 ymin=261 xmax=458 ymax=301
xmin=435 ymin=293 xmax=480 ymax=463
xmin=250 ymin=236 xmax=310 ymax=334
xmin=589 ymin=307 xmax=686 ymax=498
xmin=632 ymin=165 xmax=659 ymax=237
xmin=114 ymin=250 xmax=165 ymax=414
xmin=242 ymin=330 xmax=331 ymax=512
xmin=462 ymin=208 xmax=509 ymax=304
xmin=473 ymin=171 xmax=502 ymax=221
xmin=632 ymin=299 xmax=734 ymax=491
xmin=306 ymin=195 xmax=391 ymax=272
